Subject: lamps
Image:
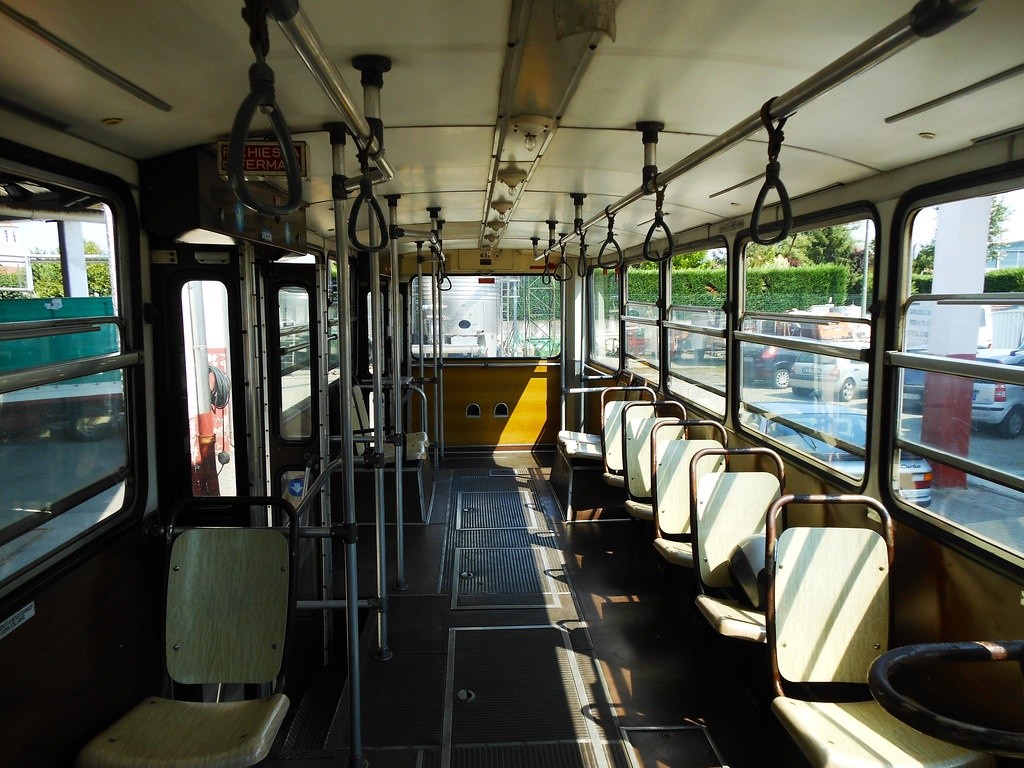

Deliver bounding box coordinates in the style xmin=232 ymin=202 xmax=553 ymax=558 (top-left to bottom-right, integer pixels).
xmin=499 ymin=173 xmax=527 ymax=195
xmin=483 ymin=235 xmax=499 ymax=248
xmin=488 ymin=222 xmax=505 ymax=238
xmin=516 ymin=123 xmax=546 ymax=152
xmin=491 ymin=201 xmax=514 ymax=220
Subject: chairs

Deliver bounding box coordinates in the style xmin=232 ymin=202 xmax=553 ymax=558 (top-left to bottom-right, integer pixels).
xmin=81 ymin=522 xmax=290 ymax=766
xmin=339 ymin=382 xmax=432 ymax=465
xmin=652 ymin=419 xmax=729 ymax=569
xmin=557 ymin=369 xmax=648 ymax=459
xmin=593 ymin=386 xmax=659 ymax=489
xmin=688 ymin=446 xmax=786 ymax=645
xmin=765 ymin=494 xmax=999 ymax=768
xmin=621 ymin=400 xmax=689 ymax=522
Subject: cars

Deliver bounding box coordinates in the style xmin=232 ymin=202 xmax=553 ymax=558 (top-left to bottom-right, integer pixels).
xmin=903 ymin=345 xmax=928 ymax=409
xmin=788 ymin=341 xmax=871 ymax=401
xmin=738 ymin=335 xmax=820 ymax=389
xmin=971 ymin=347 xmax=1024 ymax=438
